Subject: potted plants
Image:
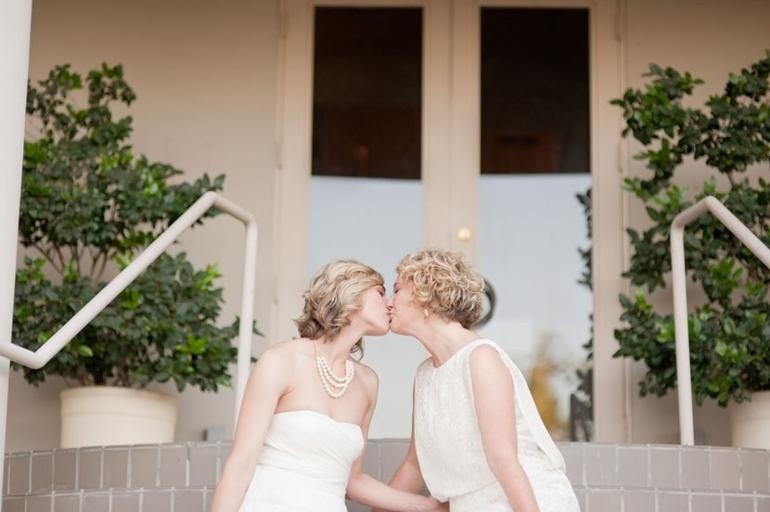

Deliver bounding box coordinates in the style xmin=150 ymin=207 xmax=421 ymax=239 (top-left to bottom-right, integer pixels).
xmin=10 ymin=59 xmax=267 ymax=451
xmin=604 ymin=44 xmax=770 ymax=452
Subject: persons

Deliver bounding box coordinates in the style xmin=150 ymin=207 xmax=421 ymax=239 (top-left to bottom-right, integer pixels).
xmin=372 ymin=250 xmax=580 ymax=512
xmin=212 ymin=258 xmax=449 ymax=511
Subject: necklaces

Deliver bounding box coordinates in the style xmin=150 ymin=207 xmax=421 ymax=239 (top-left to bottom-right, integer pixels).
xmin=315 ymin=341 xmax=359 ymax=399
